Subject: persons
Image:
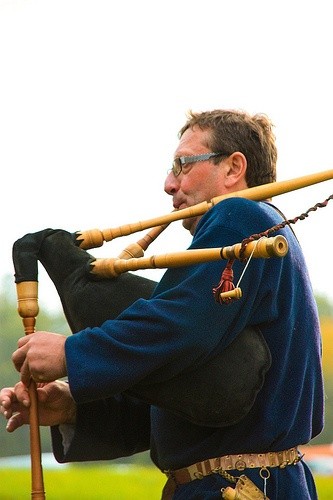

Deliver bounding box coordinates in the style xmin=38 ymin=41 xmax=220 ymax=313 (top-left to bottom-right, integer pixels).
xmin=0 ymin=111 xmax=324 ymax=500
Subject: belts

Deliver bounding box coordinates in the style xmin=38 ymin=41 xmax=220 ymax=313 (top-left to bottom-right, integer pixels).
xmin=161 ymin=446 xmax=301 ymax=500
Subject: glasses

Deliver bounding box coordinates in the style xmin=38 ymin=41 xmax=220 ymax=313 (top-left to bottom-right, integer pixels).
xmin=166 ymin=149 xmax=233 ymax=182
